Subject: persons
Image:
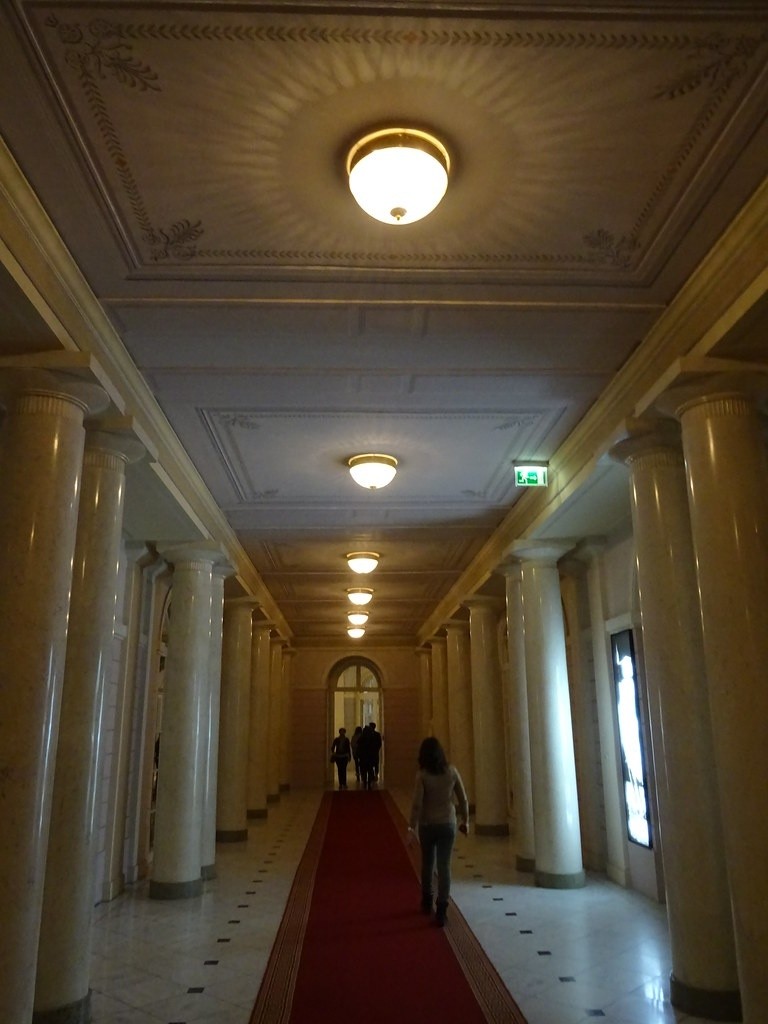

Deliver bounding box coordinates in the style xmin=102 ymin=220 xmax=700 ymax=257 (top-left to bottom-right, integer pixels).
xmin=351 ymin=722 xmax=381 ymax=789
xmin=331 ymin=728 xmax=351 ymax=788
xmin=411 ymin=736 xmax=469 ymax=926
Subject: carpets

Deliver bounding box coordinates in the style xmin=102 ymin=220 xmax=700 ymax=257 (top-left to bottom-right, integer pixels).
xmin=248 ymin=789 xmax=529 ymax=1024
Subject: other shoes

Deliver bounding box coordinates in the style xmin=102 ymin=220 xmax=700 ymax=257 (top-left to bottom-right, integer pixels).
xmin=356 ymin=774 xmax=378 ymax=789
xmin=339 ymin=784 xmax=347 ymax=788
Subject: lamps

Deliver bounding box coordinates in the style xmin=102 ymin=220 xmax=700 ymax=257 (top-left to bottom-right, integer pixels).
xmin=345 ymin=124 xmax=452 ymax=226
xmin=347 ymin=626 xmax=366 ymax=638
xmin=347 ymin=587 xmax=374 ymax=606
xmin=346 ymin=551 xmax=380 ymax=575
xmin=348 ymin=453 xmax=398 ymax=490
xmin=347 ymin=610 xmax=369 ymax=625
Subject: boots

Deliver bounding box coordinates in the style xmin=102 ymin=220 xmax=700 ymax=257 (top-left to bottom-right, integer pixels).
xmin=421 ymin=896 xmax=433 ymax=913
xmin=436 ymin=901 xmax=448 ymax=925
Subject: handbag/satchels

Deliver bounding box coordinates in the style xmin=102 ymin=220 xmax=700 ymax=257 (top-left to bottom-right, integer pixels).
xmin=331 ymin=753 xmax=336 ymax=762
